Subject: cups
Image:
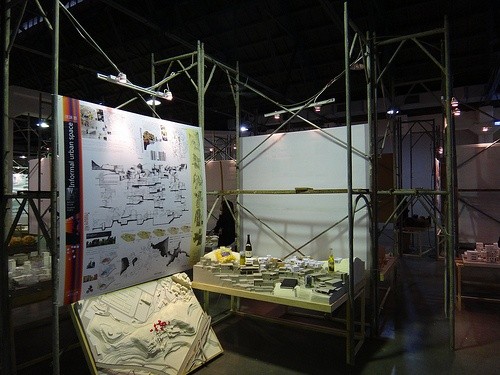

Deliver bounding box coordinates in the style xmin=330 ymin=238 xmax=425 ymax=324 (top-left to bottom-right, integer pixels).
xmin=240 ymin=251 xmax=245 ymax=266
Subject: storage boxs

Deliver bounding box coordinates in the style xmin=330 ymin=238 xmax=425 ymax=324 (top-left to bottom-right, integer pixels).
xmin=467 ymin=242 xmax=500 ymax=262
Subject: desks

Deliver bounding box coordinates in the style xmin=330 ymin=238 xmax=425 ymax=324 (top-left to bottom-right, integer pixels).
xmin=191 ymin=281 xmax=365 ymax=366
xmin=9 ymin=280 xmax=80 ymax=375
xmin=401 ymin=227 xmax=435 ymax=257
xmin=380 ymin=252 xmax=400 ymax=310
xmin=455 ymin=256 xmax=500 ymax=312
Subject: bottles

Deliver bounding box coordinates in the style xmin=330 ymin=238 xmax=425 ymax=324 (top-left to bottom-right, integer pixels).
xmin=245 ymin=234 xmax=253 ymax=267
xmin=327 ymin=248 xmax=335 ymax=274
